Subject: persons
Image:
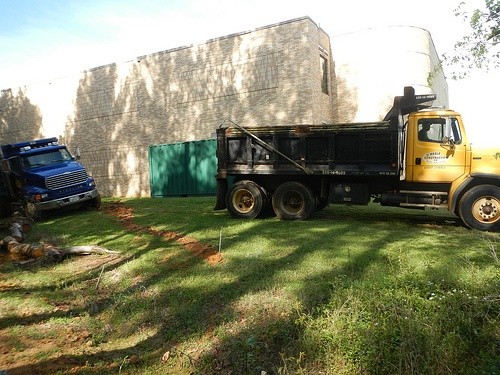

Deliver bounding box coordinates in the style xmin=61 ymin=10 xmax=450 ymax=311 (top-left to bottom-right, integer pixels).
xmin=419 ymin=121 xmax=434 ymax=141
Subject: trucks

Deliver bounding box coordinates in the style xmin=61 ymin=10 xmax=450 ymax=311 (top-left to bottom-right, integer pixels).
xmin=214 ymin=87 xmax=500 ymax=232
xmin=0 ymin=138 xmax=102 ymax=222
